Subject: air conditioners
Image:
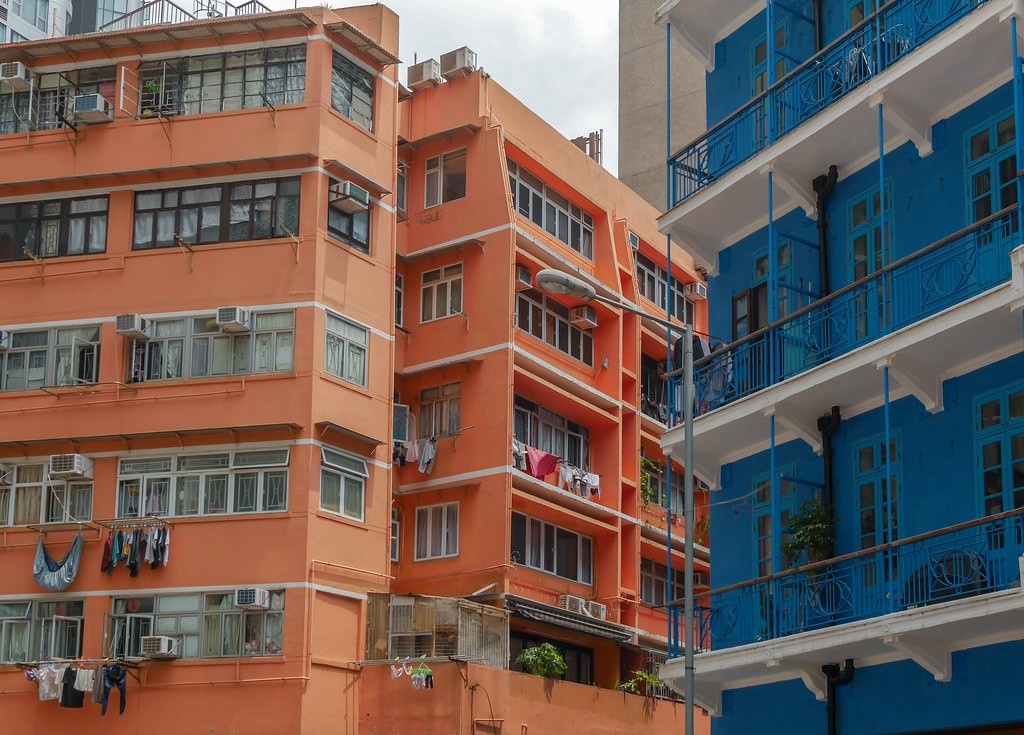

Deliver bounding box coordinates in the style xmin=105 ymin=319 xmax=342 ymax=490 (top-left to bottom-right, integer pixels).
xmin=141 ymin=635 xmax=177 ymax=654
xmin=217 ymin=306 xmax=251 ymax=332
xmin=658 ymin=360 xmax=675 ymax=381
xmin=0 ymin=330 xmax=9 ymax=350
xmin=74 ymin=93 xmax=114 ymax=125
xmin=571 ymin=306 xmax=598 ymax=329
xmin=515 ymin=266 xmax=534 ymax=291
xmin=234 ymin=588 xmax=270 ymax=610
xmin=692 ymin=572 xmax=709 ymax=589
xmin=629 ymin=231 xmax=639 ymax=251
xmin=558 ymin=594 xmax=585 ymax=615
xmin=693 ymin=475 xmax=708 ymax=493
xmin=585 ymin=601 xmax=606 ymax=621
xmin=407 ymin=58 xmax=440 ymax=91
xmin=685 ymin=282 xmax=706 ymax=302
xmin=0 ymin=61 xmax=37 ymax=89
xmin=49 ymin=454 xmax=93 ymax=480
xmin=329 ymin=181 xmax=369 ymax=215
xmin=440 ymin=46 xmax=474 ymax=81
xmin=115 ymin=313 xmax=151 ymax=339
